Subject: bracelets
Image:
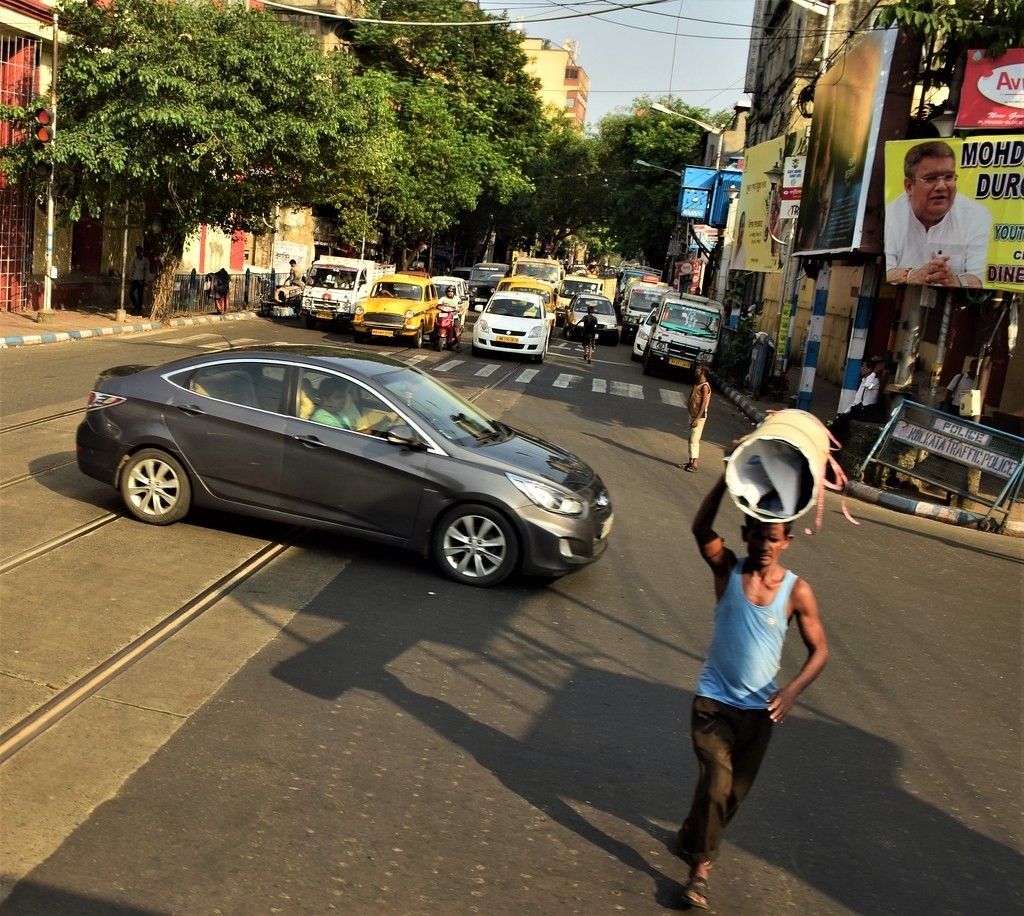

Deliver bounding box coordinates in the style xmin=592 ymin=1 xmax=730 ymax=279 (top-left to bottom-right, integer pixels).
xmin=902 ymin=266 xmax=915 ymax=283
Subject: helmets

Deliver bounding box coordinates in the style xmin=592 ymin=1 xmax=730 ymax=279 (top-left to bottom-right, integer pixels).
xmin=446 ymin=285 xmax=455 ymax=292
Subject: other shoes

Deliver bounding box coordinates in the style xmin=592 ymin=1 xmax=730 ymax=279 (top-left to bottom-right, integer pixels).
xmin=588 ymin=359 xmax=591 ymax=364
xmin=584 ymin=354 xmax=587 ymax=359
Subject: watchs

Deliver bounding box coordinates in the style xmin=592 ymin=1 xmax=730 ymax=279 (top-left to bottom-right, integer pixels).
xmin=956 ymin=274 xmax=970 ymax=288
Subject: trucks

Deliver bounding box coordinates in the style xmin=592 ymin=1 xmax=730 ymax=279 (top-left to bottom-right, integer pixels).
xmin=302 ymin=254 xmax=397 ymax=329
xmin=613 ymin=264 xmax=725 ymax=385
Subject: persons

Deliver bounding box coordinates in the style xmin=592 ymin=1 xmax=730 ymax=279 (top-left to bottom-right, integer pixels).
xmin=730 ymin=211 xmax=747 ymax=269
xmin=572 ymin=306 xmax=598 ymax=364
xmin=377 ymin=283 xmax=396 ymax=298
xmin=674 ymin=364 xmax=711 ymax=472
xmin=885 ymin=141 xmax=994 ymax=289
xmin=942 ymin=358 xmax=978 ymax=421
xmin=437 ymin=284 xmax=463 ymax=352
xmin=842 ymin=359 xmax=880 ymax=422
xmin=283 ymin=259 xmax=301 ymax=285
xmin=128 ymin=245 xmax=150 ymax=315
xmin=310 ymin=377 xmax=397 ymax=435
xmin=665 ymin=436 xmax=827 ymax=908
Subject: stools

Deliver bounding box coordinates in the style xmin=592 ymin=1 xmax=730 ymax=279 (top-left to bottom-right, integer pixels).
xmin=845 ymin=420 xmax=888 ymax=458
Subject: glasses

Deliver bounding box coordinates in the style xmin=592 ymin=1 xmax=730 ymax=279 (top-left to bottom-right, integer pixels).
xmin=908 ymin=173 xmax=958 ymax=186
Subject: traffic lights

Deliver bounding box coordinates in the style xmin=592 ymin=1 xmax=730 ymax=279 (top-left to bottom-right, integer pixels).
xmin=33 ymin=107 xmax=53 ymax=175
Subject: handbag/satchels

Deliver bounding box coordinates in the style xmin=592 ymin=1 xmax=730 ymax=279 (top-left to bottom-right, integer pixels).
xmin=851 ymin=402 xmax=868 ymax=421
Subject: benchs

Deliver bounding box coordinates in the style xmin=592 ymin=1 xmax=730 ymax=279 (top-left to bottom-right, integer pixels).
xmin=194 ymin=369 xmax=261 ymax=409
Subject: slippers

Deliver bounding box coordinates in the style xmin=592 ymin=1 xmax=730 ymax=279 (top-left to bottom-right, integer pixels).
xmin=682 ymin=842 xmax=713 ymax=870
xmin=678 ymin=463 xmax=691 ymax=468
xmin=687 ymin=465 xmax=700 ymax=473
xmin=681 ymin=876 xmax=711 ymax=909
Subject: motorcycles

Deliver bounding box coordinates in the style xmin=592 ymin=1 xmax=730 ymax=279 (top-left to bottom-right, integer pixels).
xmin=434 ymin=302 xmax=462 ymax=352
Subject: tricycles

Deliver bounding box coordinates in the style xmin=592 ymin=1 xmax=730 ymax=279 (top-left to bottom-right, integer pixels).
xmin=259 ymin=282 xmax=303 ymax=320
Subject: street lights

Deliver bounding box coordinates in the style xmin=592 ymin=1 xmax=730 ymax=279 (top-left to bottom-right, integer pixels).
xmin=633 ymin=103 xmax=723 ymax=295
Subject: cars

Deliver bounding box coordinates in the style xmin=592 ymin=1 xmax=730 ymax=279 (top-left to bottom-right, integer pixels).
xmin=74 ymin=343 xmax=616 ymax=588
xmin=555 ymin=275 xmax=619 ymax=346
xmin=451 ymin=258 xmax=565 ymax=364
xmin=351 ymin=269 xmax=470 ymax=349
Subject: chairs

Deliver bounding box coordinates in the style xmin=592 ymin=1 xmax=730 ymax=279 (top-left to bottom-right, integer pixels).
xmin=346 ymin=383 xmax=368 ymax=431
xmin=693 ymin=313 xmax=709 ymax=330
xmin=300 ymin=378 xmax=317 ymax=420
xmin=597 ymin=304 xmax=605 ymax=311
xmin=668 ymin=309 xmax=687 ymax=325
xmin=568 ymin=281 xmax=578 ymax=290
xmin=494 ymin=300 xmax=512 ymax=313
xmin=581 ymin=283 xmax=591 ymax=292
xmin=524 ymin=302 xmax=537 ymax=317
